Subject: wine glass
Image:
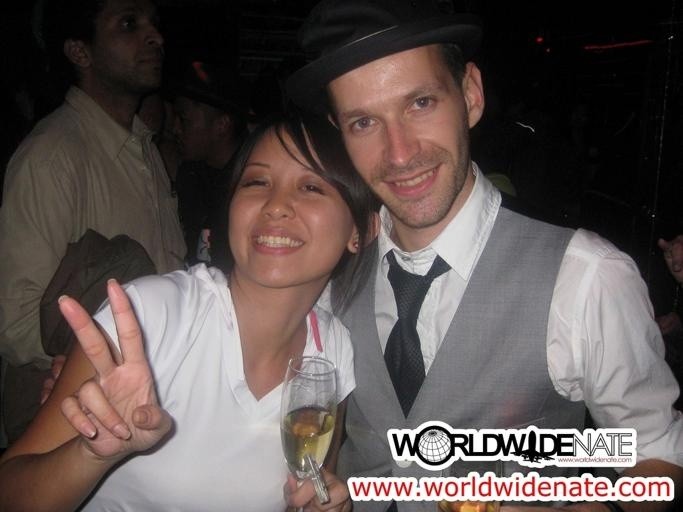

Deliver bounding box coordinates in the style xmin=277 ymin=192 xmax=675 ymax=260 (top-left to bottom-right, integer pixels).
xmin=278 ymin=353 xmax=338 ymax=511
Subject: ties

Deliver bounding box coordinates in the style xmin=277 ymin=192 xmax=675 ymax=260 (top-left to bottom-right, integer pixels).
xmin=385 ymin=253 xmax=451 ymax=417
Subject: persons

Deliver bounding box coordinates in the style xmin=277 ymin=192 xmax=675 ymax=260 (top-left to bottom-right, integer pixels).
xmin=0 ymin=0 xmax=683 ymax=512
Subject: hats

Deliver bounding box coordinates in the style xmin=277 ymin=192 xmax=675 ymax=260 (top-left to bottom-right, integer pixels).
xmin=281 ymin=0 xmax=481 ymax=117
xmin=162 ymin=67 xmax=263 ymax=127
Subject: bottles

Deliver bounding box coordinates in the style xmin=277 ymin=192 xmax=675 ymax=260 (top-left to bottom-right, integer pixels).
xmin=193 ymin=220 xmax=216 ymax=268
